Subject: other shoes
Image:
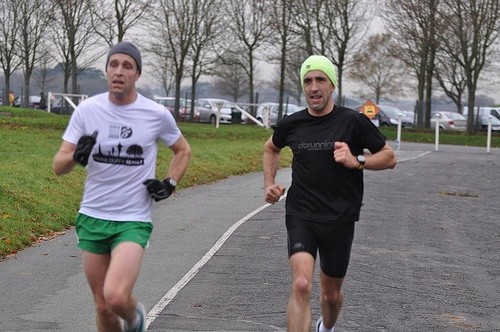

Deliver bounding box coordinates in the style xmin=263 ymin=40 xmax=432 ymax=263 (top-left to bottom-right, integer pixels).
xmin=316 ymin=317 xmax=335 ymax=332
xmin=123 ymin=304 xmax=146 ymax=331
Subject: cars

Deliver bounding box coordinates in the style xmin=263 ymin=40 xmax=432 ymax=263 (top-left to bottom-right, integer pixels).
xmin=353 ymin=104 xmax=468 ymax=133
xmin=151 ymin=95 xmax=307 ymax=127
xmin=12 ymin=95 xmax=86 ymax=115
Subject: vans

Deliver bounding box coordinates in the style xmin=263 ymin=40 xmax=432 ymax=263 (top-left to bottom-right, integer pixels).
xmin=463 ymin=106 xmax=500 ymax=131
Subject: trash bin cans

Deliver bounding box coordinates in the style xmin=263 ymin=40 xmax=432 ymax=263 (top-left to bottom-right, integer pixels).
xmin=231 ymin=108 xmax=241 ymax=124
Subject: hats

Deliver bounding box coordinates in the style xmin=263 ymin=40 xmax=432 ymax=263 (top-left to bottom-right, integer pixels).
xmin=105 ymin=42 xmax=142 ymax=74
xmin=300 ymin=55 xmax=336 ymax=93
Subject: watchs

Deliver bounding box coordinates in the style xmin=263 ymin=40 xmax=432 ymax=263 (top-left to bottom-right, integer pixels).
xmin=356 ymin=155 xmax=366 ymax=169
xmin=163 ymin=178 xmax=177 ymax=189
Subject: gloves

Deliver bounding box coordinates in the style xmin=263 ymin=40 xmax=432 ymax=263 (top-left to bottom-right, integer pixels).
xmin=72 ymin=135 xmax=96 ymax=167
xmin=143 ymin=178 xmax=173 ymax=202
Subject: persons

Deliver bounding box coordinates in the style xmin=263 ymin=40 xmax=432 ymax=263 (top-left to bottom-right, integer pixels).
xmin=9 ymin=90 xmax=15 ymax=107
xmin=263 ymin=55 xmax=398 ymax=332
xmin=52 ymin=42 xmax=191 ymax=332
xmin=39 ymin=91 xmax=47 ymax=109
